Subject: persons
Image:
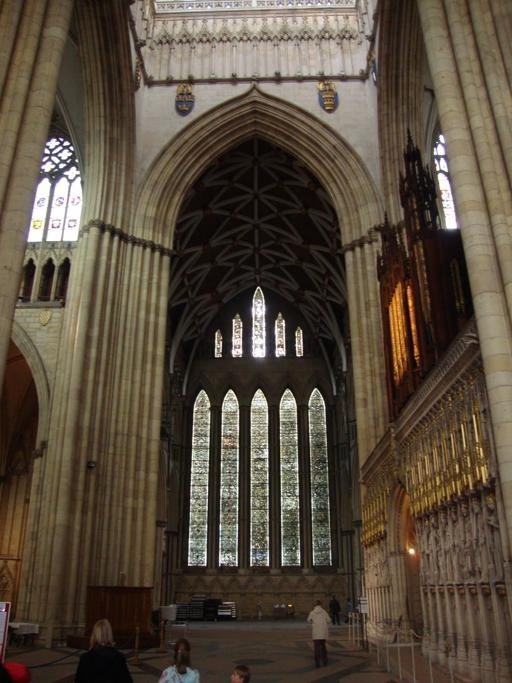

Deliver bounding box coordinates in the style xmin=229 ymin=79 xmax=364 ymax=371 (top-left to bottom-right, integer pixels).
xmin=157 ymin=639 xmax=200 ymax=683
xmin=230 ymin=664 xmax=250 ymax=683
xmin=74 ymin=617 xmax=134 ymax=683
xmin=305 ymin=599 xmax=333 ymax=669
xmin=344 ymin=597 xmax=353 ymax=625
xmin=329 ymin=594 xmax=342 ymax=626
